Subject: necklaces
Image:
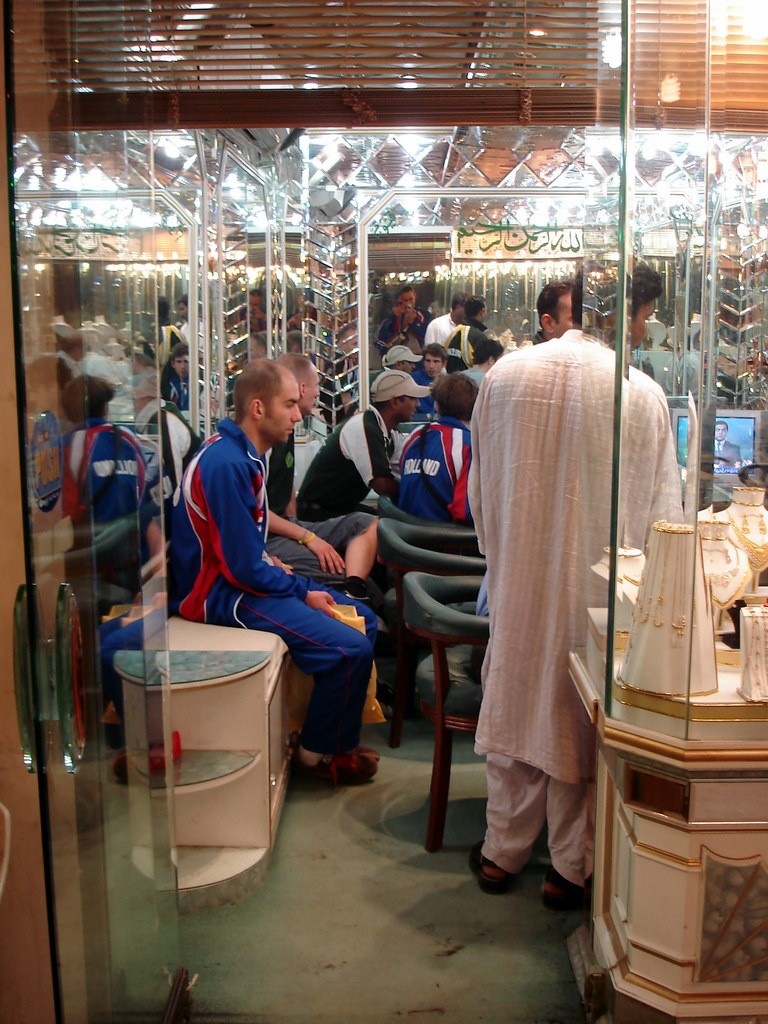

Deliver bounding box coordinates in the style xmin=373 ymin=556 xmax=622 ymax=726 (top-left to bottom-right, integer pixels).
xmin=593 ymin=500 xmax=768 ymax=700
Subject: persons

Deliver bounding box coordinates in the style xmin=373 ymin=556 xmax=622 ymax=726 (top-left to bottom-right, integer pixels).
xmin=714 ymin=420 xmax=742 ymax=469
xmin=49 ymin=295 xmax=296 ymax=741
xmin=467 ymin=256 xmax=685 ymax=917
xmin=171 ymin=292 xmax=703 ymax=786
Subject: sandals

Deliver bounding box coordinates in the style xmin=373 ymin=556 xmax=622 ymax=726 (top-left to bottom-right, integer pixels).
xmin=469 ymin=840 xmax=514 ymax=895
xmin=542 ymin=865 xmax=584 ymax=912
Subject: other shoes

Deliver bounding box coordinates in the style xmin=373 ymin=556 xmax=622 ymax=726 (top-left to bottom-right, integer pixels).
xmin=378 ymin=682 xmax=416 ymax=720
xmin=290 ymin=742 xmax=380 ymax=788
xmin=343 ymin=577 xmax=374 ymax=603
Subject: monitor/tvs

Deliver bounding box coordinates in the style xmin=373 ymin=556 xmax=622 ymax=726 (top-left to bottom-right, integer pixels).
xmin=670 ymin=408 xmax=762 ymax=482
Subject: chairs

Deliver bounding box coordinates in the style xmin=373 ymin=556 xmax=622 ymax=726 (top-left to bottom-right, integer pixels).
xmin=376 ymin=492 xmax=487 ymax=853
xmin=30 ymin=513 xmax=137 ymax=731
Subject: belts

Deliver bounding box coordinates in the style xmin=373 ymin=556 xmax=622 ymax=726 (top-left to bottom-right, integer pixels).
xmin=296 ymin=502 xmax=321 ymax=510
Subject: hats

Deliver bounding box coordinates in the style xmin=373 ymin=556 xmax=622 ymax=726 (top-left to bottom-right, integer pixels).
xmin=370 ymin=369 xmax=431 ymax=402
xmin=384 ymin=345 xmax=423 ymax=366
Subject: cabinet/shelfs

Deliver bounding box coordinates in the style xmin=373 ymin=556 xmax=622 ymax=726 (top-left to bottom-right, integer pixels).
xmin=112 ymin=618 xmax=287 ymax=910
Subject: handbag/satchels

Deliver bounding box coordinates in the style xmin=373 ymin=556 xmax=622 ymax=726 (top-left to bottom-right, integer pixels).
xmin=283 ymin=603 xmax=386 ymax=731
